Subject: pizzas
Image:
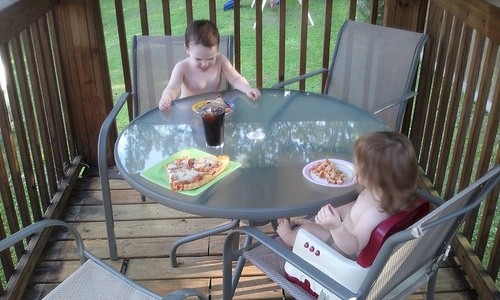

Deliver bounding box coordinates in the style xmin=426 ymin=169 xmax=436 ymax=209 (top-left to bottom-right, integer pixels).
xmin=165 ymin=155 xmax=230 ymax=191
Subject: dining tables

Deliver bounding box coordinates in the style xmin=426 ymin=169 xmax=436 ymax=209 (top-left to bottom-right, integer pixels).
xmin=115 ymin=87 xmax=398 ymax=299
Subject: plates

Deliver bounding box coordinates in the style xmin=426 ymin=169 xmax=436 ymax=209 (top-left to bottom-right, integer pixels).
xmin=192 ymin=101 xmax=233 ymax=115
xmin=140 ymin=147 xmax=242 ymax=197
xmin=302 ymin=158 xmax=358 ymax=187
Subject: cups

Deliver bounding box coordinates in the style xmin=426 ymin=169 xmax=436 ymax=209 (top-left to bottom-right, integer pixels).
xmin=192 ymin=99 xmax=234 ymax=149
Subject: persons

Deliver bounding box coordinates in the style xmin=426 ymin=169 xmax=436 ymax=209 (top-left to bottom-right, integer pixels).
xmin=275 ymin=131 xmax=420 ymax=260
xmin=159 ymin=19 xmax=260 ymax=112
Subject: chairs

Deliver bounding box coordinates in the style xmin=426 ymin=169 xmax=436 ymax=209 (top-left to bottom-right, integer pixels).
xmin=222 ymin=164 xmax=500 ymax=300
xmin=99 ymin=35 xmax=234 ymax=261
xmin=0 ymin=220 xmax=208 ymax=300
xmin=270 ymin=19 xmax=430 ymax=132
